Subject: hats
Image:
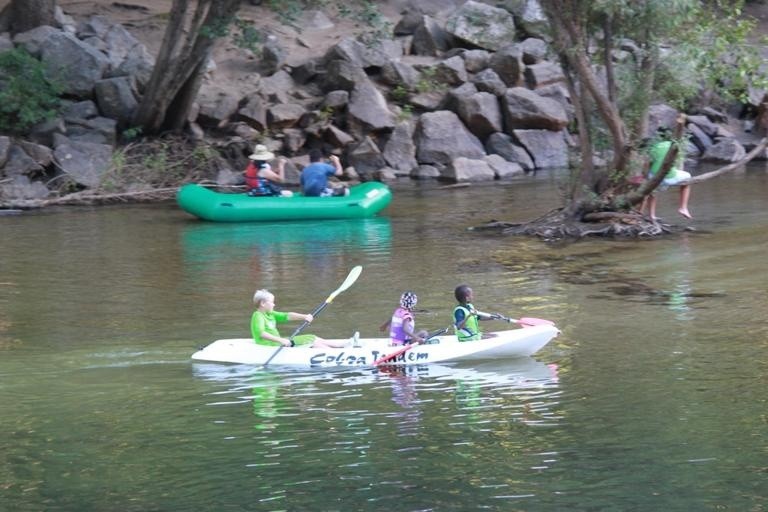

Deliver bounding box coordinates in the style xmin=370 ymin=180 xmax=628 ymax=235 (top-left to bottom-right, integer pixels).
xmin=248 ymin=144 xmax=275 ymax=161
xmin=400 ymin=292 xmax=417 ymax=309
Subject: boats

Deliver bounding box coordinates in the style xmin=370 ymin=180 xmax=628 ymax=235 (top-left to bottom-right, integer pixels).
xmin=188 ymin=315 xmax=561 ymax=367
xmin=186 ymin=357 xmax=564 ymax=392
xmin=181 ymin=219 xmax=394 ymax=254
xmin=175 ymin=176 xmax=393 ymax=217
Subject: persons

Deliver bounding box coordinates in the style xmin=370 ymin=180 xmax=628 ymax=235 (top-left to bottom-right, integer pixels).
xmin=451 ymin=285 xmax=503 ymax=343
xmin=300 ymin=149 xmax=351 ymax=197
xmin=243 ymin=144 xmax=294 ymax=198
xmin=250 ymin=288 xmax=360 ymax=350
xmin=380 ymin=290 xmax=440 ymax=346
xmin=626 ymin=137 xmax=662 ymax=221
xmin=647 ymin=126 xmax=693 ymax=221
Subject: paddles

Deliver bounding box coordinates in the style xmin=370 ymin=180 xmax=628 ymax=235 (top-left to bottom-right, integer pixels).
xmin=363 ymin=323 xmax=449 ymax=372
xmin=470 ymin=307 xmax=556 ymax=329
xmin=251 ymin=265 xmax=362 ymax=372
